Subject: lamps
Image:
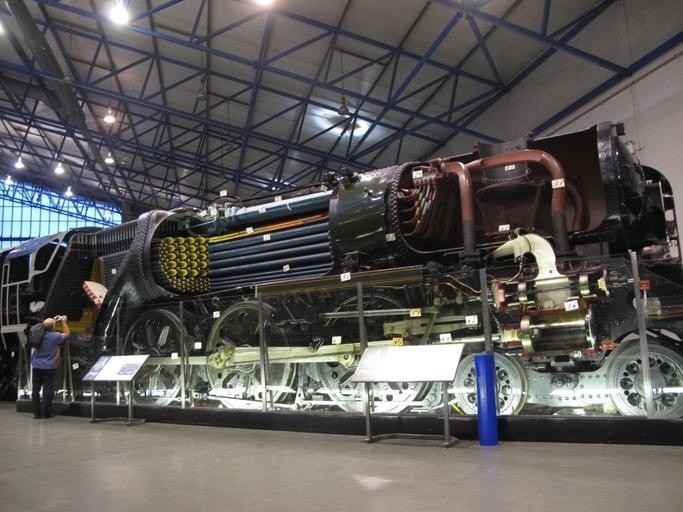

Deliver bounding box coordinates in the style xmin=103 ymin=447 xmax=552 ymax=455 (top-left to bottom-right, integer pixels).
xmin=63 ymin=24 xmax=75 ymax=83
xmin=52 ymin=161 xmax=64 ymax=174
xmin=225 ymin=98 xmax=236 ymax=152
xmin=14 ymin=155 xmax=24 ymax=168
xmin=337 ymin=49 xmax=349 ymax=115
xmin=101 ymin=68 xmax=116 ymax=124
xmin=107 ymin=1 xmax=131 ymax=24
xmin=196 ymin=39 xmax=208 ymax=101
xmin=65 ymin=186 xmax=72 ymax=196
xmin=105 ymin=151 xmax=114 ymax=164
xmin=4 ymin=174 xmax=13 ymax=186
xmin=219 ymin=129 xmax=228 ymax=197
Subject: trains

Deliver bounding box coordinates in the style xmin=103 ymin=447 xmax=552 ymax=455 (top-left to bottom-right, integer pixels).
xmin=0 ymin=119 xmax=682 ymax=420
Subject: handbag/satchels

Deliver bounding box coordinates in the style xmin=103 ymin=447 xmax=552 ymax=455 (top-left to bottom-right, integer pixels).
xmin=27 ymin=322 xmax=46 ymax=348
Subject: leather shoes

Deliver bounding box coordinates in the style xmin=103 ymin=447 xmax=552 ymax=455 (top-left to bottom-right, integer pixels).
xmin=45 ymin=412 xmax=55 ymax=418
xmin=34 ymin=410 xmax=41 ymax=418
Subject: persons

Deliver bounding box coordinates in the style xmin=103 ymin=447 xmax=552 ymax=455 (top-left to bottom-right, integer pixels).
xmin=32 ymin=315 xmax=71 ymax=418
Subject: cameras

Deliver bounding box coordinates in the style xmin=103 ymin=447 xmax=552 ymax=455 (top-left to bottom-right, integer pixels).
xmin=58 ymin=316 xmax=64 ymax=320
